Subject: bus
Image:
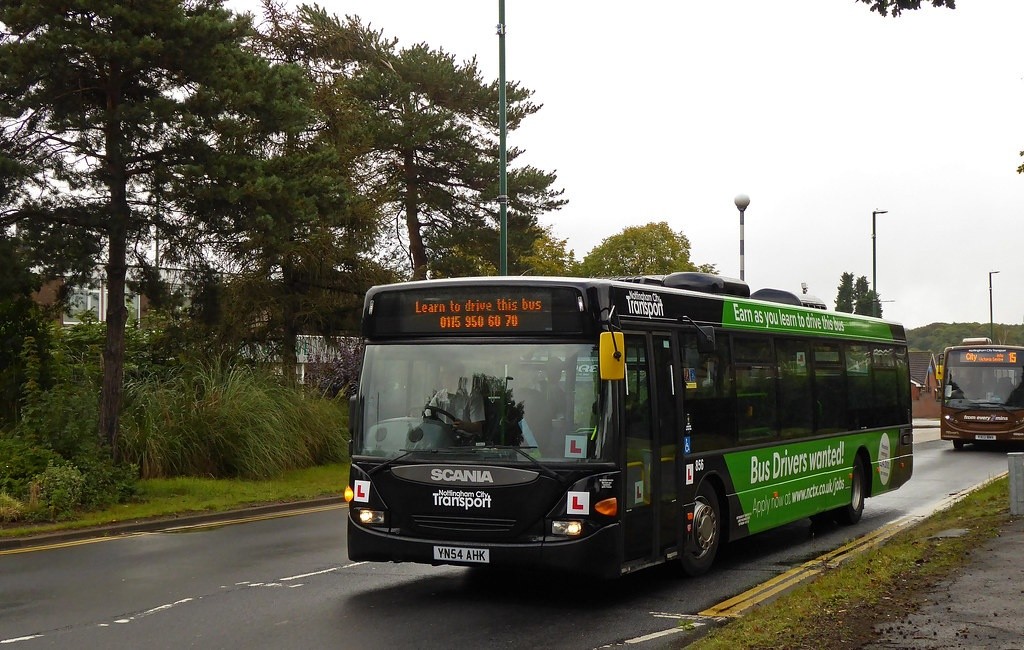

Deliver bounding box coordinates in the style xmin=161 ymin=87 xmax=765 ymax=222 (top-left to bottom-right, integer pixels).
xmin=934 ymin=337 xmax=1024 ymax=449
xmin=343 ymin=271 xmax=912 ymax=579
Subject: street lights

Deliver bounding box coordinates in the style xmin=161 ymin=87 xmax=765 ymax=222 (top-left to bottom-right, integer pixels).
xmin=989 ymin=270 xmax=1000 ymax=345
xmin=872 ymin=208 xmax=889 ymax=319
xmin=734 ymin=192 xmax=750 ymax=282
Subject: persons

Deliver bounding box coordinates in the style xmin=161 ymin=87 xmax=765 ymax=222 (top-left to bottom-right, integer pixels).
xmin=424 ymin=362 xmax=485 ymax=445
